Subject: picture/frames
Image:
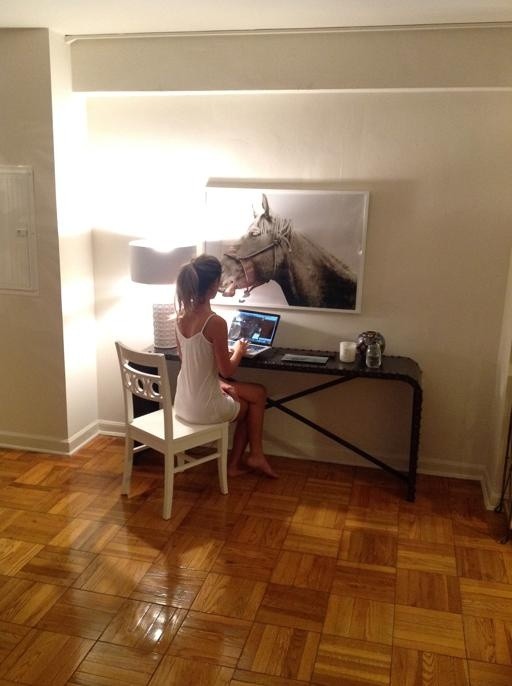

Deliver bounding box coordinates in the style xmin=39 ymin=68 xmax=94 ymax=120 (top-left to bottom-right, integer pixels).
xmin=195 ymin=184 xmax=369 ymax=316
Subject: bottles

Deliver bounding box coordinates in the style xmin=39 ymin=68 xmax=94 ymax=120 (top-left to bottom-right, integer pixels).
xmin=366 ymin=343 xmax=382 ymax=368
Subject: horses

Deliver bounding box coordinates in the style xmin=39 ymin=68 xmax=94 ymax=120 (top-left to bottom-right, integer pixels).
xmin=216 ymin=193 xmax=358 ymax=310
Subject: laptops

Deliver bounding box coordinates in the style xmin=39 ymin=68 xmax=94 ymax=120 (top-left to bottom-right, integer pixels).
xmin=228 ymin=309 xmax=281 ymax=360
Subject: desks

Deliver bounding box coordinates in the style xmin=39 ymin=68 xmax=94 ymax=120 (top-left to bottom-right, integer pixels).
xmin=128 ymin=342 xmax=422 ymax=504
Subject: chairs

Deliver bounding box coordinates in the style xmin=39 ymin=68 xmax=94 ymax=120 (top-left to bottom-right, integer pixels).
xmin=114 ymin=339 xmax=231 ymax=522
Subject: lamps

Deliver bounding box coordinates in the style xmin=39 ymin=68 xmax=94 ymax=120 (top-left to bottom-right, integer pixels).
xmin=131 ymin=236 xmax=196 ymax=348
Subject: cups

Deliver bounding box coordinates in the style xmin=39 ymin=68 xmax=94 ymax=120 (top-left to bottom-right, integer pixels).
xmin=339 ymin=341 xmax=356 ymax=363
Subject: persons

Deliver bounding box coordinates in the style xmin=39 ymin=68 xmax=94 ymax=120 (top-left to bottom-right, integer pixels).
xmin=173 ymin=253 xmax=282 ymax=480
